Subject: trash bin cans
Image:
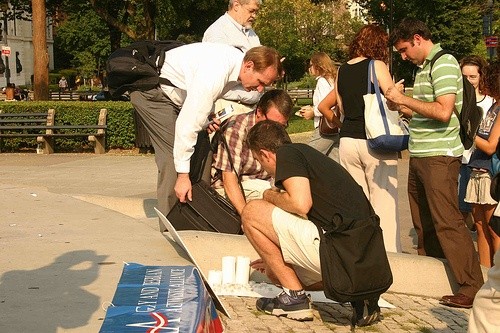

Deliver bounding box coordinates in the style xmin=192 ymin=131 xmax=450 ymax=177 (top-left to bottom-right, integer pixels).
xmin=6 ymin=83 xmax=15 ymax=100
xmin=132 ymin=108 xmax=152 ymax=149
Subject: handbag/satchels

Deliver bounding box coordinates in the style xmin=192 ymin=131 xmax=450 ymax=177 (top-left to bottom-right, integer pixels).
xmin=320 ymin=217 xmax=394 ymax=302
xmin=316 ymin=77 xmax=340 ymax=135
xmin=363 ymin=62 xmax=410 ymax=151
xmin=165 ymin=124 xmax=248 ymax=235
xmin=101 ymin=37 xmax=185 ymax=94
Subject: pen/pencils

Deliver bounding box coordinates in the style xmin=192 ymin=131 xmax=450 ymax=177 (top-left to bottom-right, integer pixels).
xmin=398 ymin=79 xmax=404 ymax=84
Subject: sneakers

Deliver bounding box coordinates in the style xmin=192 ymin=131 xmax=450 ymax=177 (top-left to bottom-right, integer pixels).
xmin=350 ymin=297 xmax=381 ymax=325
xmin=256 ymin=292 xmax=315 ymax=320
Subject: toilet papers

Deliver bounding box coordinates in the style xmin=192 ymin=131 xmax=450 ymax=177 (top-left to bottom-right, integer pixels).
xmin=207 ymin=269 xmax=221 ymax=284
xmin=221 ymin=255 xmax=235 ymax=285
xmin=235 ymin=256 xmax=250 ymax=284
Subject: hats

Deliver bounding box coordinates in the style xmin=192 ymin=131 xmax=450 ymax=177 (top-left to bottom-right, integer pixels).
xmin=62 ymin=76 xmax=66 ymax=80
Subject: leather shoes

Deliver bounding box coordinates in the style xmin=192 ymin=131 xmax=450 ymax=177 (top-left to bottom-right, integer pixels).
xmin=440 ymin=294 xmax=473 ymax=308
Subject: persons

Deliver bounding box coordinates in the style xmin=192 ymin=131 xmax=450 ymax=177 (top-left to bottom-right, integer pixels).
xmin=468 ymin=135 xmax=500 ymax=333
xmin=2 ymin=83 xmax=19 ymax=102
xmin=59 ymin=76 xmax=68 ymax=91
xmin=130 ymin=0 xmax=393 ymax=326
xmin=334 ymin=16 xmax=500 ymax=308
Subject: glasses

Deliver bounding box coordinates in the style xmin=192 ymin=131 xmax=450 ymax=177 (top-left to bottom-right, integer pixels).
xmin=264 ymin=114 xmax=289 ymax=130
xmin=307 ymin=64 xmax=312 ymax=69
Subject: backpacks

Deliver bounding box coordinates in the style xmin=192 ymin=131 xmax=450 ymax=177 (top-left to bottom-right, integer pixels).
xmin=430 ymin=50 xmax=483 ymax=149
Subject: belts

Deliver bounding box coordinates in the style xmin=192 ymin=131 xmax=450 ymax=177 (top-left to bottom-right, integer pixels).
xmin=7 ymin=99 xmax=13 ymax=100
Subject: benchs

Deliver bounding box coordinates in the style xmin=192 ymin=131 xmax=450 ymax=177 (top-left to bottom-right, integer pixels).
xmin=284 ymin=88 xmax=313 ymax=106
xmin=0 ymin=108 xmax=108 ymax=155
xmin=49 ymin=91 xmax=81 ymax=100
